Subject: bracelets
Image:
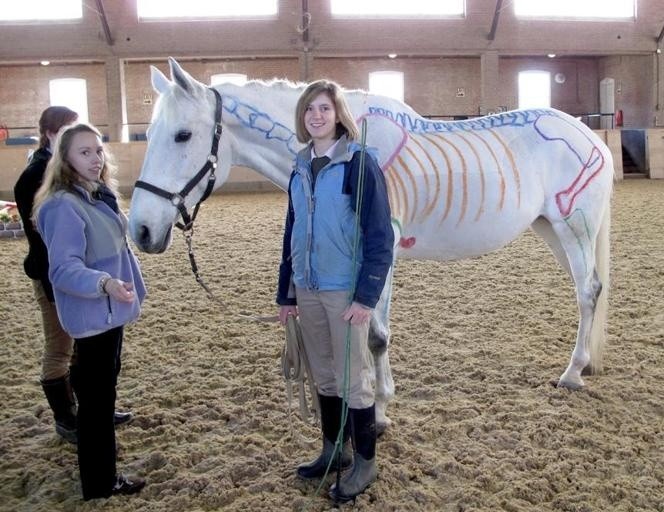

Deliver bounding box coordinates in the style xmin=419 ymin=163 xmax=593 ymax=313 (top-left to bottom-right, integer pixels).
xmin=98 ymin=277 xmax=112 ymax=296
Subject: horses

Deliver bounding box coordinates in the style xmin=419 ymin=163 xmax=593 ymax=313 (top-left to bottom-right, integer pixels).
xmin=127 ymin=55 xmax=616 ymax=440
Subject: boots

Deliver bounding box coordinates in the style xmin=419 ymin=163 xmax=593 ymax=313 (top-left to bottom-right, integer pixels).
xmin=113 ymin=410 xmax=134 ymax=426
xmin=327 ymin=402 xmax=378 ymax=501
xmin=40 ymin=370 xmax=79 ymax=444
xmin=296 ymin=393 xmax=353 ymax=480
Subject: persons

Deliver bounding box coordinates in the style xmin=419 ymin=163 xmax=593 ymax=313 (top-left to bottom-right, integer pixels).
xmin=29 ymin=122 xmax=149 ymax=501
xmin=274 ymin=79 xmax=395 ymax=503
xmin=13 ymin=106 xmax=134 ymax=446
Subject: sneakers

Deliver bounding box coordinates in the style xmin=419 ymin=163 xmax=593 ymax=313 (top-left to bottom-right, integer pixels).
xmin=110 ymin=471 xmax=147 ymax=494
